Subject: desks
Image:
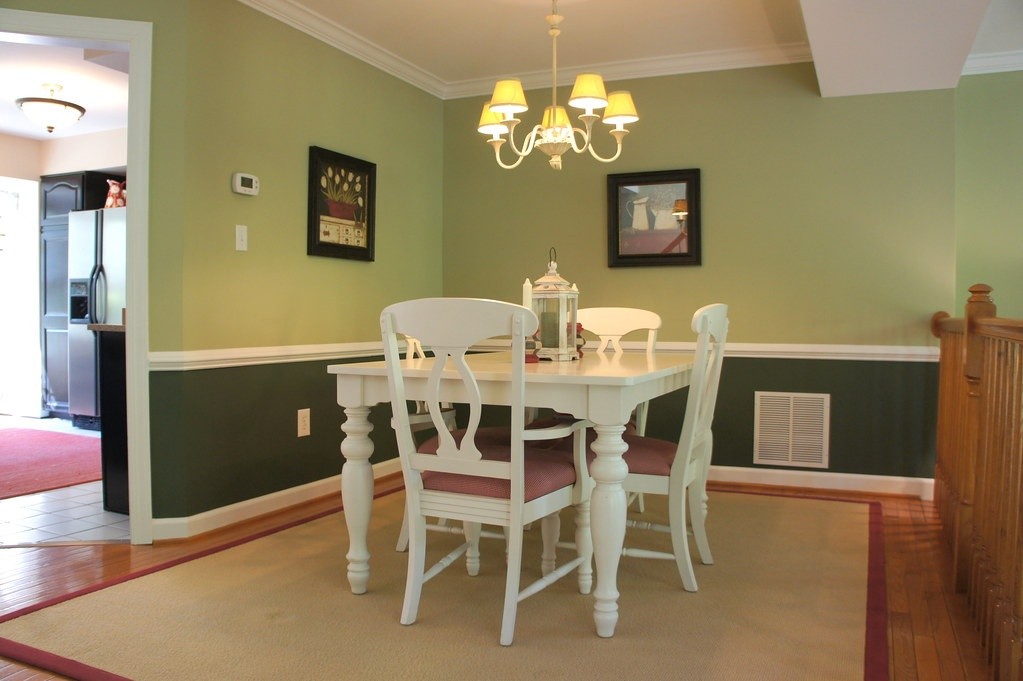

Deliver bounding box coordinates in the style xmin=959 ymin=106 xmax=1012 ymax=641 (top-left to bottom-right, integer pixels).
xmin=327 ymin=350 xmax=694 ymax=637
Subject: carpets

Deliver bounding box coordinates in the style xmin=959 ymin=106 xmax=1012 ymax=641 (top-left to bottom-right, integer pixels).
xmin=0 ymin=425 xmax=102 ymax=503
xmin=0 ymin=487 xmax=891 ymax=681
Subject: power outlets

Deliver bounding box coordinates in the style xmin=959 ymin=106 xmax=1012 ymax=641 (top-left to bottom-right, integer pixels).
xmin=298 ymin=408 xmax=310 ymax=438
xmin=235 ymin=225 xmax=248 ymax=252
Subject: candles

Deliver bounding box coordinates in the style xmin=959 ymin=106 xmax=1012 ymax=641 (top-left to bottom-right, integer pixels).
xmin=569 ymin=282 xmax=577 ymax=324
xmin=523 ymin=277 xmax=532 ymax=312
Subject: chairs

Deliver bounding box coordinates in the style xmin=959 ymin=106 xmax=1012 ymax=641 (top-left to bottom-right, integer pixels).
xmin=380 ymin=297 xmax=591 ymax=646
xmin=390 ymin=303 xmax=729 ymax=591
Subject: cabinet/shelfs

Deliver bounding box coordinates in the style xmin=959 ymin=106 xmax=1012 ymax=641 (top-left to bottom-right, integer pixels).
xmin=38 ymin=171 xmax=126 ymax=419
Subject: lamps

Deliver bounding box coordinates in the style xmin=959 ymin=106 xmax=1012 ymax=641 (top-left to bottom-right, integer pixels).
xmin=12 ymin=82 xmax=86 ymax=134
xmin=673 ymin=199 xmax=688 ymax=236
xmin=532 ymin=246 xmax=579 ymax=361
xmin=477 ymin=0 xmax=639 ymax=169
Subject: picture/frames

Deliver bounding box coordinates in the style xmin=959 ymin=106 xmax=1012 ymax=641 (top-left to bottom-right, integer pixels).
xmin=607 ymin=168 xmax=702 ymax=267
xmin=306 ymin=145 xmax=377 ymax=262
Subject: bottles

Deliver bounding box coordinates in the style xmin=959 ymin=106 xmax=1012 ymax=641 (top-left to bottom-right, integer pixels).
xmin=525 ymin=328 xmax=542 ymax=363
xmin=567 ymin=323 xmax=586 ymax=360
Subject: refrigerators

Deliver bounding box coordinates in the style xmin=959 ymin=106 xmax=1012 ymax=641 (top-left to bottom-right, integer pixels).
xmin=67 ymin=205 xmax=126 ymax=431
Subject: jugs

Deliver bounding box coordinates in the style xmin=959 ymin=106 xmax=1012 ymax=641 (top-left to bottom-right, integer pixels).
xmin=104 ymin=180 xmax=127 ymax=208
